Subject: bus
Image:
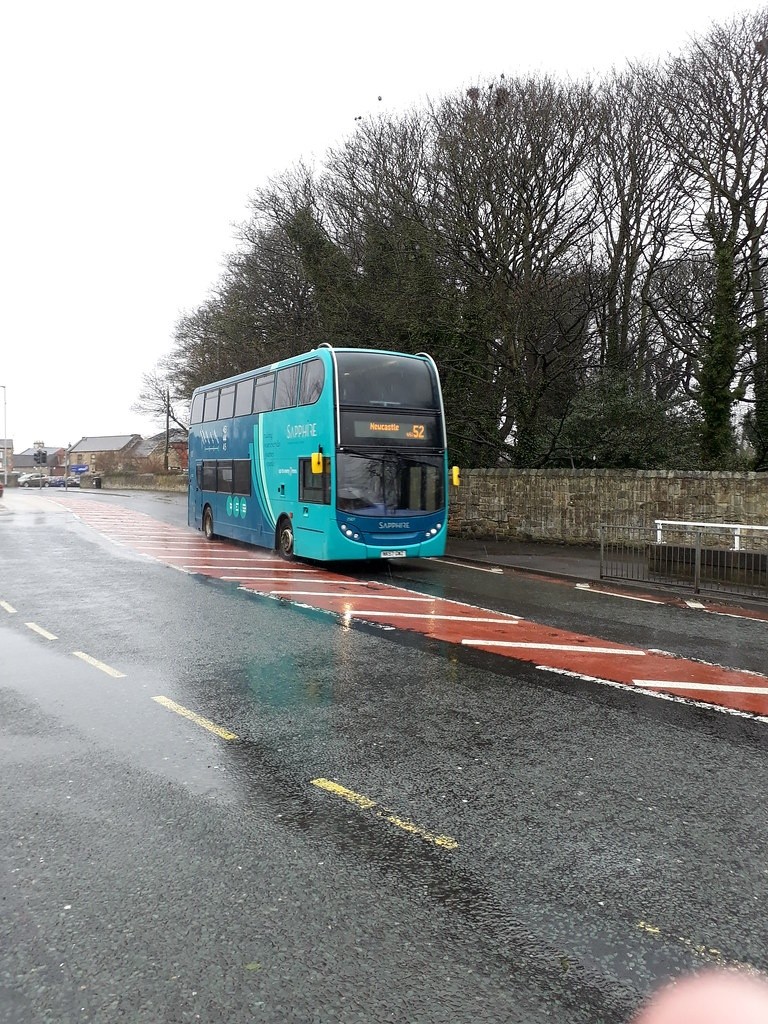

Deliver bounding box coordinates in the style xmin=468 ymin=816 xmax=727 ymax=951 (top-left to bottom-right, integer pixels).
xmin=186 ymin=342 xmax=465 ymax=564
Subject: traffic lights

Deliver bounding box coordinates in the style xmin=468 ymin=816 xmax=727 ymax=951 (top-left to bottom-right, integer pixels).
xmin=33 ymin=452 xmax=39 ymax=464
xmin=39 ymin=450 xmax=47 ymax=463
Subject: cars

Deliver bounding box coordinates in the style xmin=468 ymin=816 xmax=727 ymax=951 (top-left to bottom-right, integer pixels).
xmin=48 ymin=475 xmax=80 ymax=487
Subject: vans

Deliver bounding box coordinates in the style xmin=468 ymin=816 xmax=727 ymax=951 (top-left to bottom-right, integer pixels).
xmin=17 ymin=473 xmax=50 ymax=487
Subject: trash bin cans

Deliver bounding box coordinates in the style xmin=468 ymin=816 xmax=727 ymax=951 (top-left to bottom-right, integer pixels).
xmin=92 ymin=477 xmax=101 ymax=489
